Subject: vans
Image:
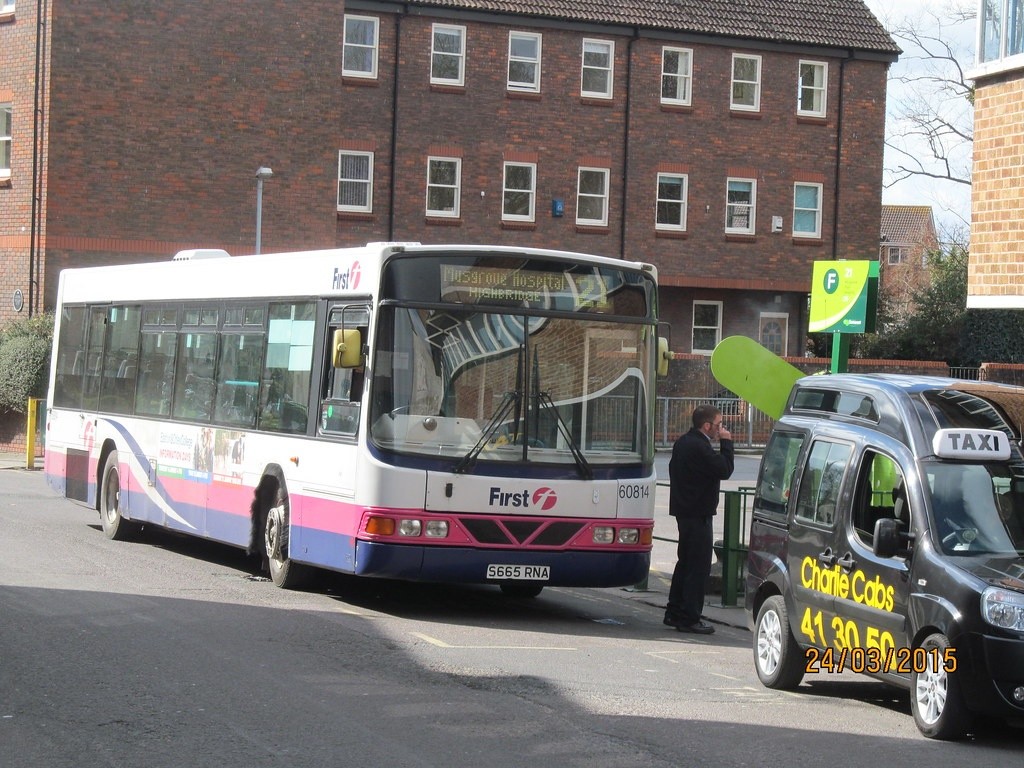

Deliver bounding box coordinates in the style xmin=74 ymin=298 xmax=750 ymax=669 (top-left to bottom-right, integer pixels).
xmin=745 ymin=373 xmax=1024 ymax=743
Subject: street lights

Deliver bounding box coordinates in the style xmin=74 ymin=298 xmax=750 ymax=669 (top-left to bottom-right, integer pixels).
xmin=254 ymin=167 xmax=274 ymax=254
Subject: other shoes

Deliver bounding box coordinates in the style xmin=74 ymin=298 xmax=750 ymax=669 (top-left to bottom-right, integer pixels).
xmin=676 ymin=620 xmax=715 ymax=634
xmin=662 ymin=611 xmax=678 ymax=626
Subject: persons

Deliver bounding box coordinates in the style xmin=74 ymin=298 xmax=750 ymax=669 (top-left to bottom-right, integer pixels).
xmin=664 ymin=404 xmax=734 ymax=635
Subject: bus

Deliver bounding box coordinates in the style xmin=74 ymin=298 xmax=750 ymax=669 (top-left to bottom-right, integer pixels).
xmin=44 ymin=242 xmax=676 ymax=602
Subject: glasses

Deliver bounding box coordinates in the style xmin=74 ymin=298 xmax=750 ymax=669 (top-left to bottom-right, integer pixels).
xmin=706 ymin=421 xmax=723 ymax=429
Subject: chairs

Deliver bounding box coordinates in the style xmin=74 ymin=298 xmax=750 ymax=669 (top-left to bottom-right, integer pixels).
xmin=61 ymin=344 xmax=167 ymax=379
xmin=892 ymin=480 xmax=909 ymax=526
